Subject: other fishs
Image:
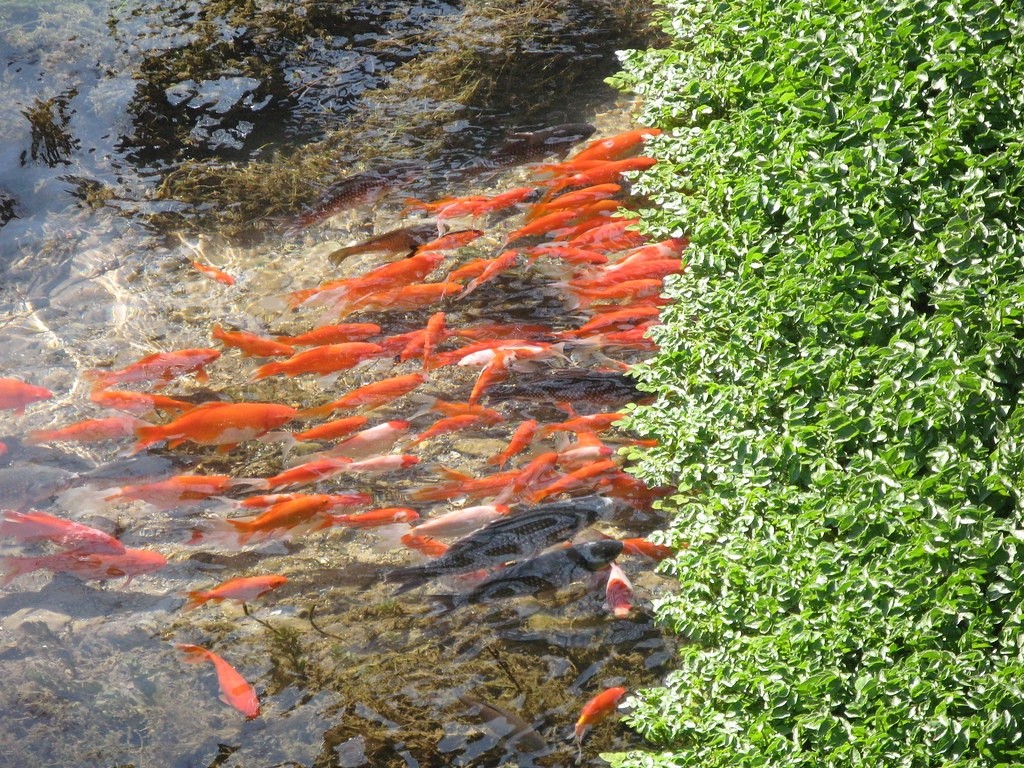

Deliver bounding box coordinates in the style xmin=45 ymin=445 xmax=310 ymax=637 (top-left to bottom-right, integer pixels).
xmin=1 ymin=129 xmax=688 ymax=745
